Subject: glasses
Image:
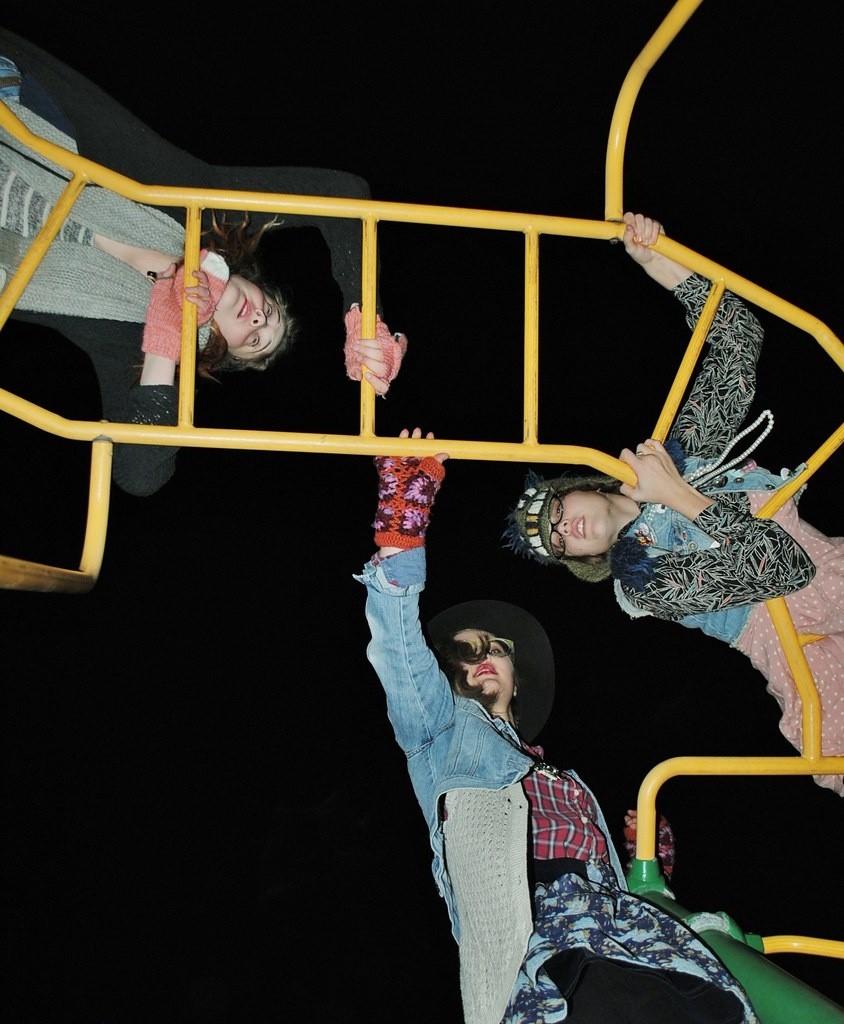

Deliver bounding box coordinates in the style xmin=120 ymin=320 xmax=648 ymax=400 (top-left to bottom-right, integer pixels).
xmin=462 ymin=638 xmax=516 ymax=667
xmin=549 ymin=486 xmax=579 ymax=561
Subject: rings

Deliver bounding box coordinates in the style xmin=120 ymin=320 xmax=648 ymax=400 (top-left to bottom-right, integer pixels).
xmin=636 ymin=452 xmax=644 ymax=458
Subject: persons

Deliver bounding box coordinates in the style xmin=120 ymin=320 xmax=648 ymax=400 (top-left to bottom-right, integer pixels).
xmin=512 ymin=212 xmax=844 ymax=800
xmin=352 ymin=426 xmax=758 ymax=1024
xmin=0 ymin=56 xmax=408 ymax=495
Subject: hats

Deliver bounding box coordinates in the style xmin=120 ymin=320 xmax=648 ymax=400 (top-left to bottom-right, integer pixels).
xmin=512 ymin=476 xmax=616 ymax=583
xmin=422 ymin=599 xmax=556 ymax=744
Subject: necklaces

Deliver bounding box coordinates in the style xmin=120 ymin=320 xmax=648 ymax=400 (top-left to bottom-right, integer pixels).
xmin=686 ymin=409 xmax=774 ymax=488
